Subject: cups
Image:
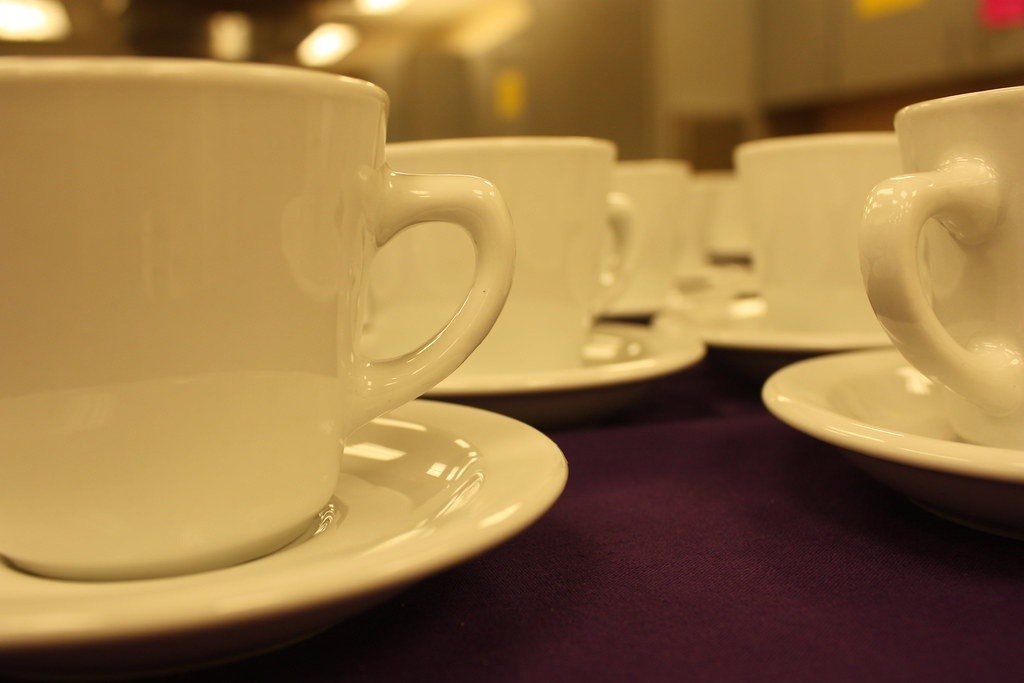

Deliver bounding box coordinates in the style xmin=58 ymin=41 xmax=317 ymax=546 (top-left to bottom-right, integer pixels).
xmin=0 ymin=55 xmax=517 ymax=582
xmin=859 ymin=86 xmax=1024 ymax=453
xmin=611 ymin=159 xmax=686 ymax=317
xmin=387 ymin=136 xmax=616 ymax=375
xmin=682 ymin=173 xmax=743 ymax=308
xmin=730 ymin=131 xmax=903 ymax=327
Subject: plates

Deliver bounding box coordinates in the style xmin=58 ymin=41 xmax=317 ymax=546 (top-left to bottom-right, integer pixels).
xmin=0 ymin=398 xmax=570 ymax=654
xmin=652 ymin=308 xmax=893 ymax=351
xmin=760 ymin=348 xmax=1024 ymax=483
xmin=421 ymin=322 xmax=707 ymax=395
